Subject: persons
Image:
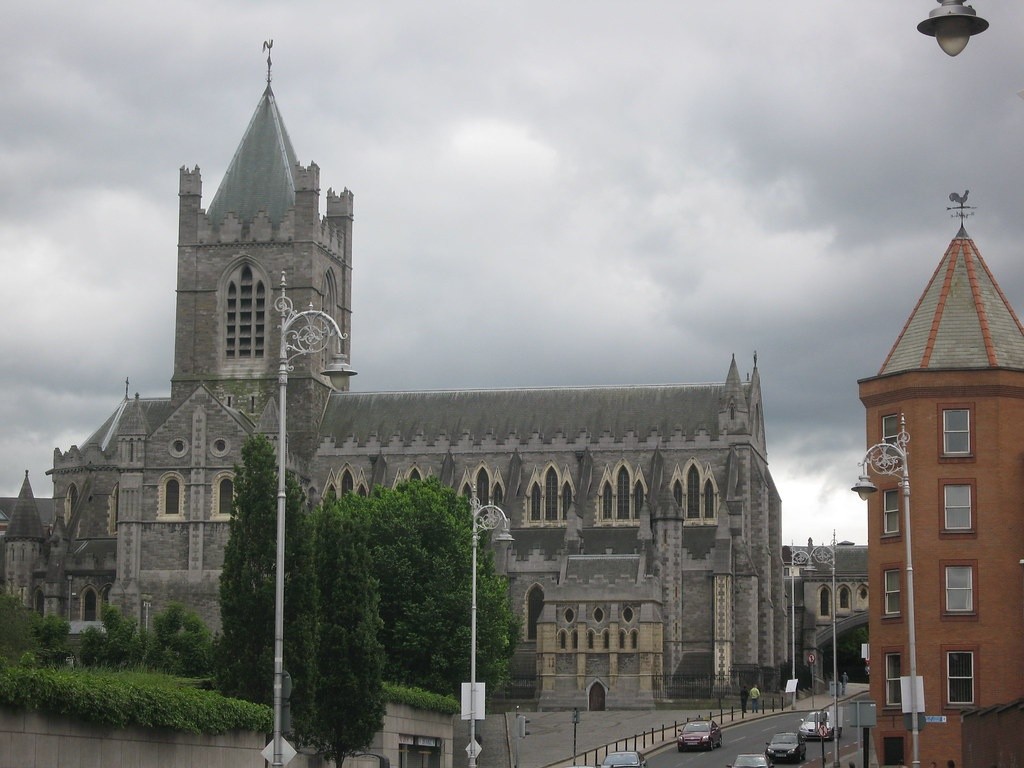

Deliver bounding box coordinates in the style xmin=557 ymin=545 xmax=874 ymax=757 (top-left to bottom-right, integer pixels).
xmin=740 ymin=686 xmax=749 ymax=713
xmin=841 ymin=672 xmax=848 ymax=695
xmin=749 ymin=684 xmax=760 ymax=713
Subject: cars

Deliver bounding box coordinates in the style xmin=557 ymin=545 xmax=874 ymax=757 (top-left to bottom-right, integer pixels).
xmin=595 ymin=751 xmax=648 ymax=768
xmin=798 ymin=711 xmax=842 ymax=741
xmin=765 ymin=731 xmax=807 ymax=764
xmin=677 ymin=720 xmax=722 ymax=751
xmin=727 ymin=753 xmax=775 ymax=768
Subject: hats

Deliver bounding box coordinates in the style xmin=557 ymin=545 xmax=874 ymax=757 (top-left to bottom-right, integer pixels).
xmin=844 ymin=672 xmax=846 ymax=674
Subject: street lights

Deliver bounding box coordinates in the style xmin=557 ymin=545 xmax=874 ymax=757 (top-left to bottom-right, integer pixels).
xmin=817 ymin=527 xmax=839 ymax=768
xmin=791 ymin=539 xmax=818 ymax=710
xmin=271 ymin=268 xmax=359 ymax=768
xmin=851 ymin=409 xmax=923 ymax=768
xmin=468 ymin=492 xmax=517 ymax=768
